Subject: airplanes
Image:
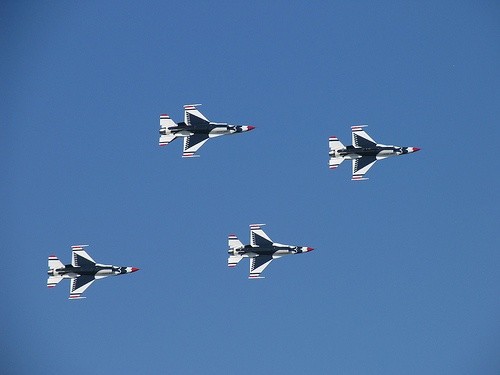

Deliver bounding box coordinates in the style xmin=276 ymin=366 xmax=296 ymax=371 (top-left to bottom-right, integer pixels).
xmin=329 ymin=123 xmax=421 ymax=181
xmin=228 ymin=223 xmax=316 ymax=280
xmin=46 ymin=243 xmax=143 ymax=300
xmin=157 ymin=103 xmax=255 ymax=159
